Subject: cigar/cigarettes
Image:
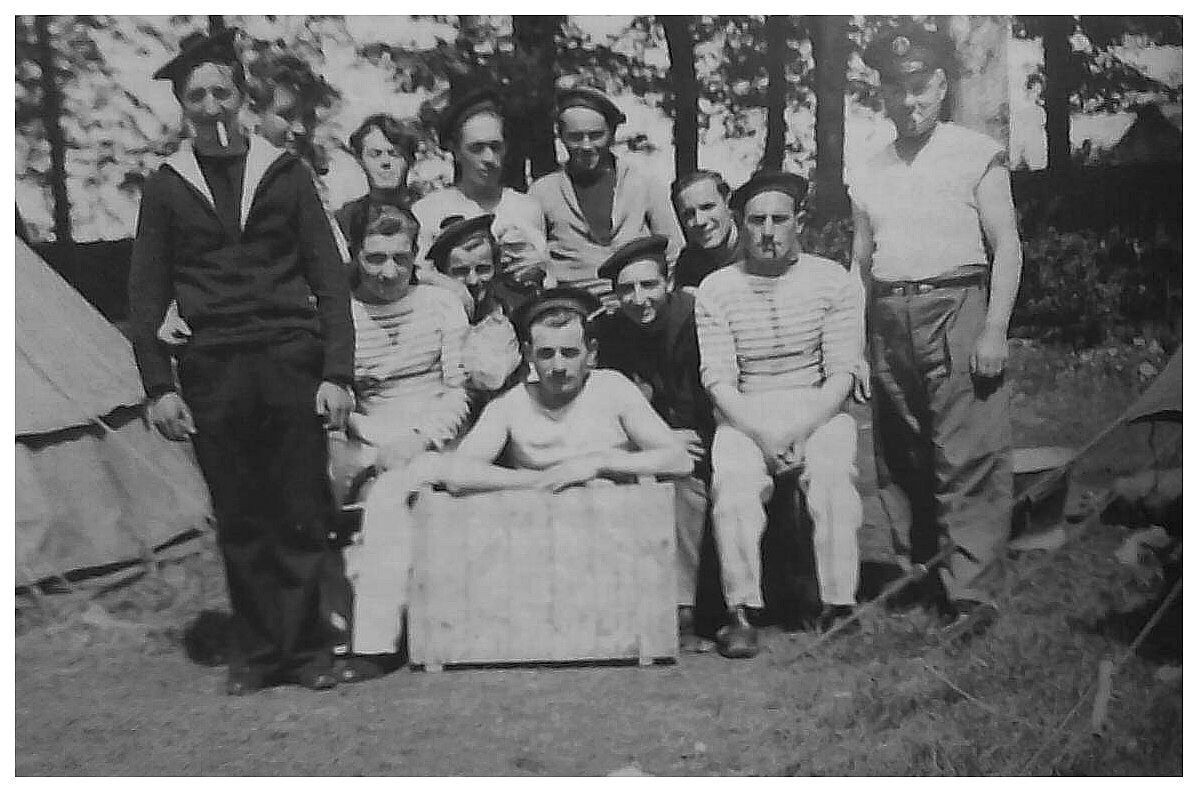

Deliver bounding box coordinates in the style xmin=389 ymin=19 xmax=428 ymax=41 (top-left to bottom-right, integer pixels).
xmin=216 ymin=119 xmax=227 ymax=147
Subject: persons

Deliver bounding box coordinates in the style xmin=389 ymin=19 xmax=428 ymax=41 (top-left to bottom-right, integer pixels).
xmin=838 ymin=28 xmax=1020 ymax=632
xmin=128 ymin=28 xmax=357 ymax=696
xmin=326 ymin=89 xmax=873 ymax=677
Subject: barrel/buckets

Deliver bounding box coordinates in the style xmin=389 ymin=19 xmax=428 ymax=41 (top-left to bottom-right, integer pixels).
xmin=1010 ymin=444 xmax=1076 ymax=539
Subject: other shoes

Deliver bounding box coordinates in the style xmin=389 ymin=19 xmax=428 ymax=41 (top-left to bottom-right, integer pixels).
xmin=227 ymin=660 xmax=275 ymax=694
xmin=885 ymin=585 xmax=940 ymax=621
xmin=951 ymin=603 xmax=992 ymax=637
xmin=339 ymin=657 xmax=376 ymax=680
xmin=822 ymin=604 xmax=862 ymax=636
xmin=284 ymin=657 xmax=339 ymax=690
xmin=715 ymin=608 xmax=760 ymax=658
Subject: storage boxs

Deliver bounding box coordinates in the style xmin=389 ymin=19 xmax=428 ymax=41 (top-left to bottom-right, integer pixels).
xmin=404 ymin=482 xmax=680 ymax=673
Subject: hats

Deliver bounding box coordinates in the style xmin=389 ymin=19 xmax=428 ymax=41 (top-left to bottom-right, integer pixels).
xmin=152 ymin=27 xmax=238 ymax=81
xmin=728 ymin=169 xmax=809 ymax=210
xmin=439 ymin=82 xmax=506 ymax=149
xmin=862 ymin=25 xmax=956 ymax=73
xmin=509 ymin=287 xmax=603 ymax=331
xmin=556 ymin=86 xmax=626 ymax=130
xmin=426 ymin=213 xmax=494 ymax=263
xmin=598 ymin=233 xmax=669 ymax=282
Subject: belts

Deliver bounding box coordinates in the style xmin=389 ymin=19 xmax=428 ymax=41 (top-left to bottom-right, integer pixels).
xmin=871 ymin=274 xmax=984 ymax=296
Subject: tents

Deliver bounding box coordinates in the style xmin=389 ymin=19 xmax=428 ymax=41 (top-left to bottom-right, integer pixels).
xmin=15 ymin=236 xmax=214 ymax=600
xmin=1065 ymin=342 xmax=1182 ymax=641
